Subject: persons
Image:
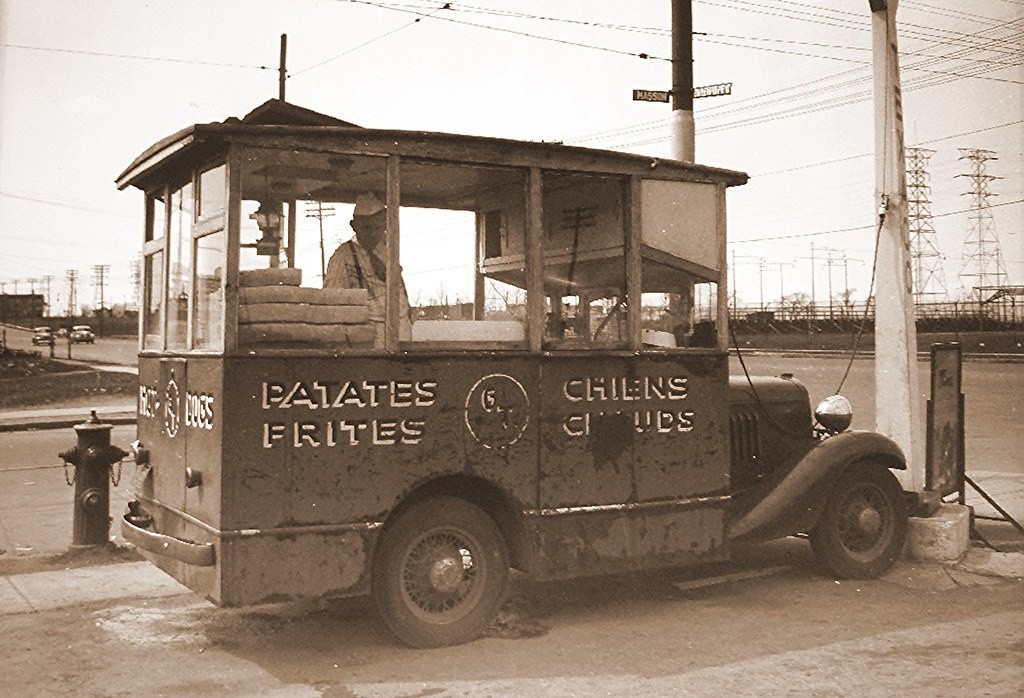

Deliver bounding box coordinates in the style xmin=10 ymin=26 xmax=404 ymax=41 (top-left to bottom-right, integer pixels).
xmin=323 ymin=189 xmax=412 ymax=350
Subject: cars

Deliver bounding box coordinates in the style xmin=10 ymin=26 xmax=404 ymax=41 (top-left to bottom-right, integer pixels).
xmin=112 ymin=119 xmax=913 ymax=650
xmin=57 ymin=328 xmax=69 ymax=338
xmin=66 ymin=325 xmax=95 ymax=345
xmin=31 ymin=326 xmax=56 ymax=346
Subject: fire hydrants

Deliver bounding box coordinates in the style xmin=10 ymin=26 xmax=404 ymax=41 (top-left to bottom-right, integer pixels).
xmin=58 ymin=410 xmax=130 ymax=556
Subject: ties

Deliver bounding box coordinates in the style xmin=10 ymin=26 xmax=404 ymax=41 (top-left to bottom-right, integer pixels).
xmin=371 ymin=250 xmax=387 ymax=282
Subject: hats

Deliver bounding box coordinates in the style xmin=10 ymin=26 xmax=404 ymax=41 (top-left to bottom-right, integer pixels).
xmin=353 ymin=192 xmax=385 ymax=216
